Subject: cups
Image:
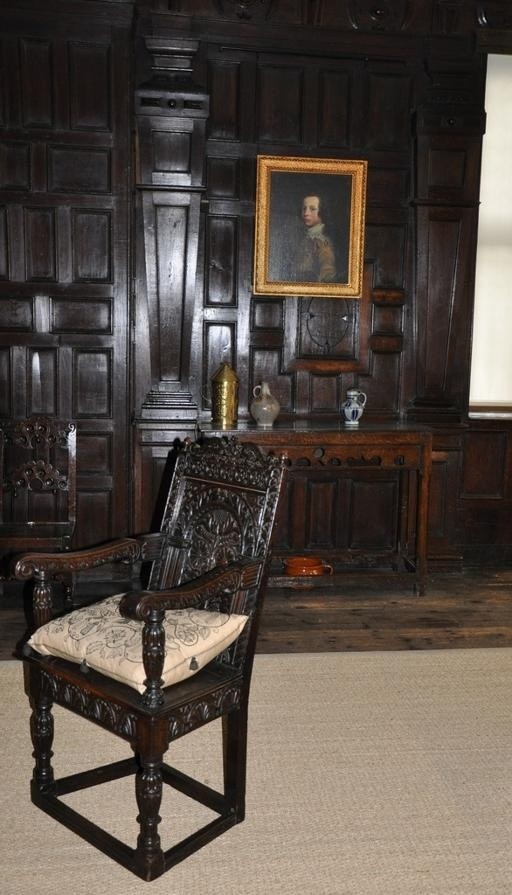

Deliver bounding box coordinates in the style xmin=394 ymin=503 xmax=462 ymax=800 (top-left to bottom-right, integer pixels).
xmin=283 ymin=556 xmax=334 ymax=577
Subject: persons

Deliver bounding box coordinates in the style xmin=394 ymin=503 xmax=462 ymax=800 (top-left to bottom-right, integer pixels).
xmin=288 ymin=187 xmax=339 ymax=283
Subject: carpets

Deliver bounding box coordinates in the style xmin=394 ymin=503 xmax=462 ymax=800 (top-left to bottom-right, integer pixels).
xmin=1 ymin=645 xmax=511 ymax=894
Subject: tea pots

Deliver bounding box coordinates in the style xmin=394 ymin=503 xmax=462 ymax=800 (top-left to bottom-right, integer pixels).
xmin=340 ymin=389 xmax=366 ymax=427
xmin=250 ymin=381 xmax=279 ymax=427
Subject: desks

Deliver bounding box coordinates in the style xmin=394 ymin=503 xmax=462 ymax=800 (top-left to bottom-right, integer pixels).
xmin=197 ymin=418 xmax=433 ymax=597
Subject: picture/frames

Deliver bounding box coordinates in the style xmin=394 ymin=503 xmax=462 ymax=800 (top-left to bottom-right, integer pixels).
xmin=253 ymin=154 xmax=370 ymax=300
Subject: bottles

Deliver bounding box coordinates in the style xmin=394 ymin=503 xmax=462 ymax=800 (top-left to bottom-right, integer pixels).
xmin=211 ymin=363 xmax=238 ymax=429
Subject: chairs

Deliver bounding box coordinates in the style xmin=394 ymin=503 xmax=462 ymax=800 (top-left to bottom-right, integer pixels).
xmin=0 ymin=414 xmax=78 ymax=611
xmin=8 ymin=435 xmax=287 ymax=881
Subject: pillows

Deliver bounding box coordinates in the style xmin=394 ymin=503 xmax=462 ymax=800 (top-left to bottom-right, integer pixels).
xmin=26 ymin=591 xmax=251 ymax=699
